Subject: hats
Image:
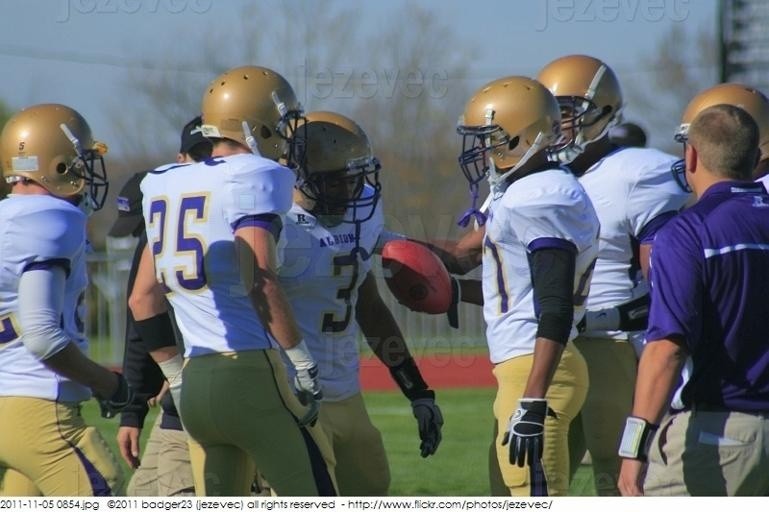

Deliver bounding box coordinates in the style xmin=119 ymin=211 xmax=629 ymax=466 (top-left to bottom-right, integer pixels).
xmin=180 ymin=117 xmax=212 ymax=151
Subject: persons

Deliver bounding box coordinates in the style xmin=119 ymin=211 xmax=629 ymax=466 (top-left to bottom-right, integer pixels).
xmin=108 ymin=168 xmax=184 ymax=431
xmin=450 ymin=74 xmax=601 ymax=496
xmin=128 ymin=64 xmax=339 ymax=496
xmin=374 ymin=54 xmax=692 ymax=495
xmin=276 ymin=109 xmax=445 ymax=496
xmin=116 ymin=115 xmax=337 ymax=497
xmin=672 ymin=82 xmax=769 ymax=212
xmin=616 ymin=109 xmax=769 ymax=495
xmin=0 ymin=102 xmax=136 ymax=495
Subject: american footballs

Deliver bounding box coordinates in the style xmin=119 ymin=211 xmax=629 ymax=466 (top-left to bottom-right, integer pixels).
xmin=382 ymin=240 xmax=453 ymax=315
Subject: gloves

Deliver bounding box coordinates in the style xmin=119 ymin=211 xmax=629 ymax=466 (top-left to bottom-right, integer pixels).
xmin=93 ymin=370 xmax=137 ymax=419
xmin=413 ymin=397 xmax=444 ymax=458
xmin=292 ymin=366 xmax=324 ymax=429
xmin=502 ymin=396 xmax=547 ymax=468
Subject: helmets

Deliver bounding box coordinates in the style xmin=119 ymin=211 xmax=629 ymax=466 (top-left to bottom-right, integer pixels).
xmin=456 ymin=75 xmax=563 ymax=176
xmin=537 ymin=55 xmax=622 ymax=144
xmin=201 ymin=65 xmax=302 ymax=156
xmin=0 ymin=104 xmax=107 ymax=198
xmin=284 ymin=111 xmax=381 ymax=222
xmin=670 ymin=81 xmax=768 ymax=192
